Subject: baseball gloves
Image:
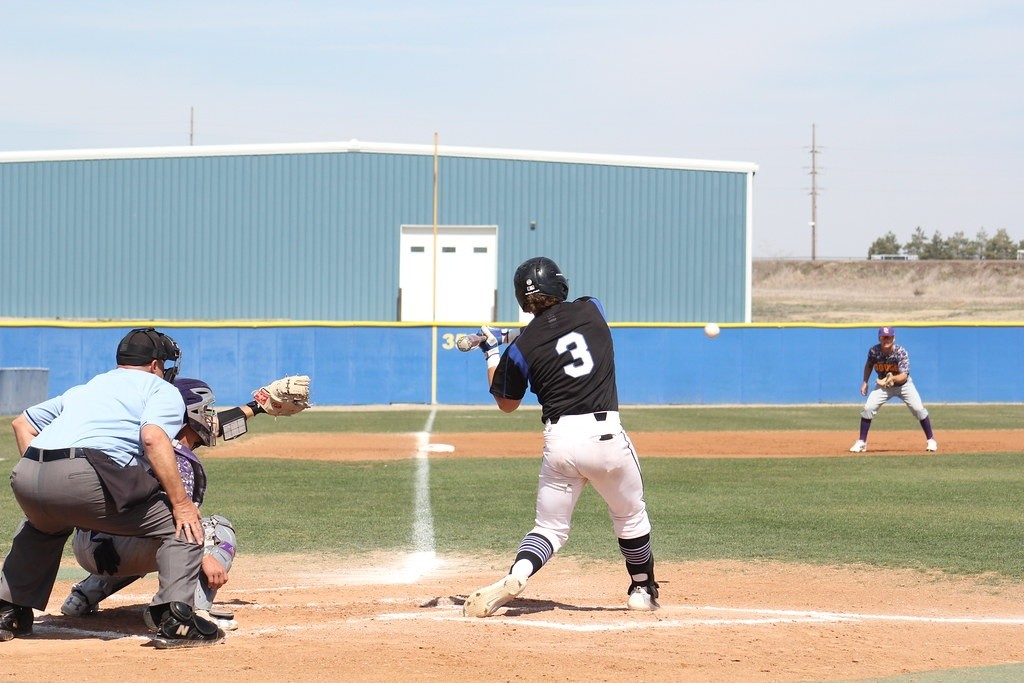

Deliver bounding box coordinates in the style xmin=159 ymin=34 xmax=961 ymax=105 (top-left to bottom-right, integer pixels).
xmin=875 ymin=372 xmax=894 ymax=388
xmin=252 ymin=375 xmax=311 ymax=416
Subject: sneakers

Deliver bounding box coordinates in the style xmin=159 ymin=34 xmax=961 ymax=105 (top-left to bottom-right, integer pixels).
xmin=926 ymin=438 xmax=937 ymax=451
xmin=627 ymin=580 xmax=660 ymax=611
xmin=0 ymin=602 xmax=34 ymax=640
xmin=850 ymin=440 xmax=866 ymax=452
xmin=60 ymin=590 xmax=99 ymax=617
xmin=463 ymin=573 xmax=528 ymax=617
xmin=154 ymin=620 xmax=225 ymax=648
xmin=194 ymin=609 xmax=238 ymax=631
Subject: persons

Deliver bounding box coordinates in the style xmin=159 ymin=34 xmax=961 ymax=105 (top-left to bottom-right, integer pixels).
xmin=461 ymin=257 xmax=659 ymax=618
xmin=63 ymin=374 xmax=314 ymax=631
xmin=848 ymin=326 xmax=938 ymax=452
xmin=0 ymin=327 xmax=226 ymax=650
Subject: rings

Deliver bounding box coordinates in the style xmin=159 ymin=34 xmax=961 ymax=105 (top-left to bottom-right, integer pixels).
xmin=183 ymin=526 xmax=190 ymax=528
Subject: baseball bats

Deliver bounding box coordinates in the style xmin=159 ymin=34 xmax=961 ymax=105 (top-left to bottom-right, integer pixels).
xmin=457 ymin=334 xmax=488 ymax=352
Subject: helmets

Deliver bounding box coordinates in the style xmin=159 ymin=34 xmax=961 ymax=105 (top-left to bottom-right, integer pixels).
xmin=170 ymin=378 xmax=216 ymax=447
xmin=513 ymin=256 xmax=568 ymax=312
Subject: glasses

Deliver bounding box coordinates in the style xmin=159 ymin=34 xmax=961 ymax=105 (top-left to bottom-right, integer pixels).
xmin=880 ymin=336 xmax=892 ymax=341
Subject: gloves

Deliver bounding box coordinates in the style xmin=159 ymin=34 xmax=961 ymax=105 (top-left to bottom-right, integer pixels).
xmin=479 ymin=326 xmax=499 ymax=359
xmin=477 ymin=324 xmax=508 ymax=346
xmin=91 ymin=533 xmax=120 ymax=576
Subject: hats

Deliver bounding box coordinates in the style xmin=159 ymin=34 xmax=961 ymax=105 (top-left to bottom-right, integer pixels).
xmin=879 ymin=327 xmax=895 ymax=336
xmin=116 ymin=329 xmax=176 ymax=366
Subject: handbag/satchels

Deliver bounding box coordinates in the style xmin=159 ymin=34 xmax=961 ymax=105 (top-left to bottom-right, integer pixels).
xmin=83 ymin=448 xmax=160 ymax=514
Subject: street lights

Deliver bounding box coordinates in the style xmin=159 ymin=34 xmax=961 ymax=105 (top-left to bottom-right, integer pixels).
xmin=809 ymin=222 xmax=816 ymax=260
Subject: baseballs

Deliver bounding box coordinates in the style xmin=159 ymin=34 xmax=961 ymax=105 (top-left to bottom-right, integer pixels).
xmin=704 ymin=323 xmax=721 ymax=338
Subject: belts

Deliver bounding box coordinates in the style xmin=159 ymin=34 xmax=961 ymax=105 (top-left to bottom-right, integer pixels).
xmin=22 ymin=447 xmax=86 ymax=462
xmin=550 ymin=412 xmax=606 ymax=424
